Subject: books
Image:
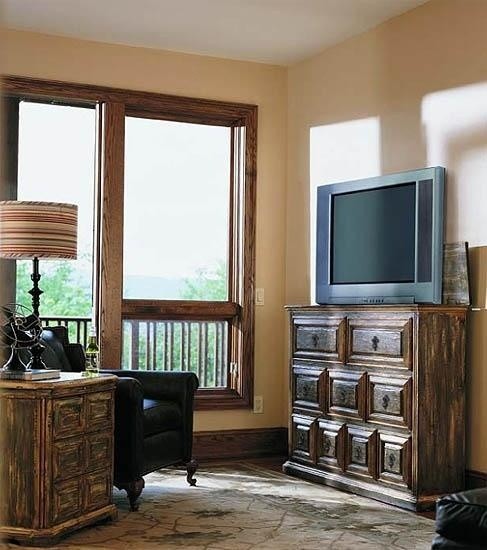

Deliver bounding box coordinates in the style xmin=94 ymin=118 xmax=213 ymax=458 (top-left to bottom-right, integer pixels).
xmin=0 ymin=368 xmax=60 ymax=381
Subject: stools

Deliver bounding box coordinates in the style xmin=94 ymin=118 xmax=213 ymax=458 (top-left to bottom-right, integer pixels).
xmin=431 ymin=488 xmax=487 ymax=549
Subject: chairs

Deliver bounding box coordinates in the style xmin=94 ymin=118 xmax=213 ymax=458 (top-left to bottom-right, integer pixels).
xmin=3 ymin=326 xmax=199 ymax=511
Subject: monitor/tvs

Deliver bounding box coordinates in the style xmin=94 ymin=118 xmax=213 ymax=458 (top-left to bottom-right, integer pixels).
xmin=315 ymin=166 xmax=446 ymax=306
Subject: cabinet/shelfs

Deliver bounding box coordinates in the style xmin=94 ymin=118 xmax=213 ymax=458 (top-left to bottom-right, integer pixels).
xmin=283 ymin=301 xmax=469 ymax=512
xmin=0 ymin=371 xmax=119 ymax=546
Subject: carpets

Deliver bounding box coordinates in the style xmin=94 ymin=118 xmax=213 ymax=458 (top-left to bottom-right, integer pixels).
xmin=0 ymin=458 xmax=436 ymax=550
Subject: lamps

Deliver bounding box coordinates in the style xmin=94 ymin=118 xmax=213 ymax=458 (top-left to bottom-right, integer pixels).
xmin=0 ymin=200 xmax=77 ymax=381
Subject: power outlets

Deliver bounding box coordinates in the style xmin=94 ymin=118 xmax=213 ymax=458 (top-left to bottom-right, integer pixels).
xmin=253 ymin=396 xmax=263 ymax=414
